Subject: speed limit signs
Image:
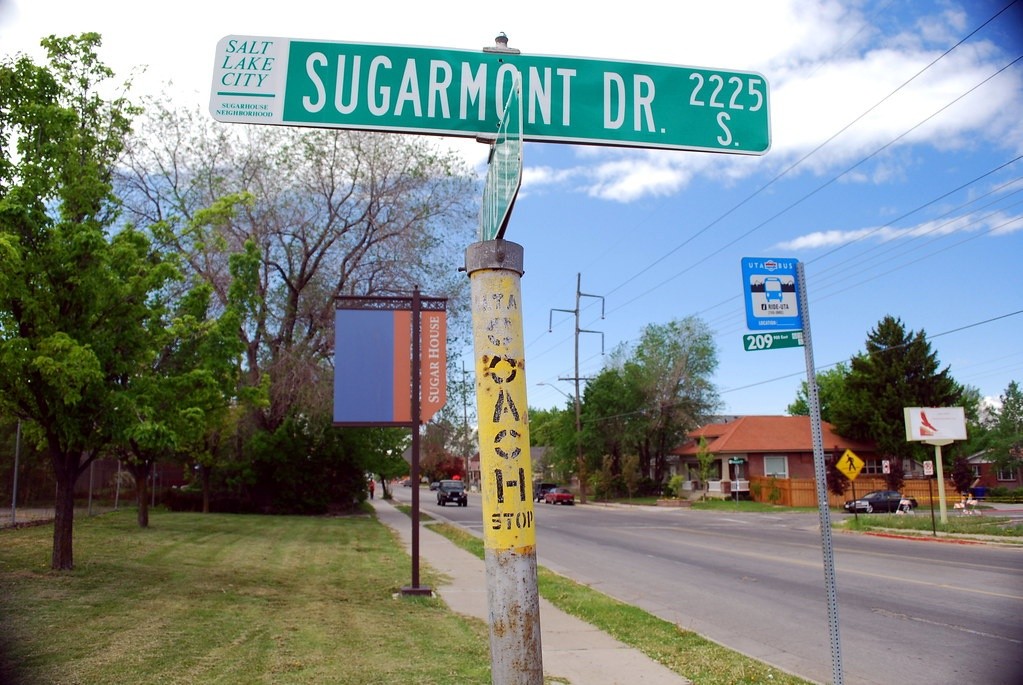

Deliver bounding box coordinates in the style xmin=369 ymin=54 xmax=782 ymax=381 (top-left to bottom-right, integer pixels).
xmin=740 ymin=256 xmax=806 ymax=351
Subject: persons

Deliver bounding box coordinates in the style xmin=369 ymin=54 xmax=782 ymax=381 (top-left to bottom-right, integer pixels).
xmin=370 ymin=476 xmax=374 ymax=499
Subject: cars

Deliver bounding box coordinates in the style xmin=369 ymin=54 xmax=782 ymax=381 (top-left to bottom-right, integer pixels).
xmin=843 ymin=487 xmax=918 ymax=514
xmin=429 ymin=480 xmax=441 ymax=492
xmin=544 ymin=486 xmax=576 ymax=505
xmin=403 ymin=481 xmax=412 ymax=487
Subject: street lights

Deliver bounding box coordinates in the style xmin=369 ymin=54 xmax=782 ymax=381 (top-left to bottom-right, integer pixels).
xmin=537 ymin=382 xmax=587 ymax=504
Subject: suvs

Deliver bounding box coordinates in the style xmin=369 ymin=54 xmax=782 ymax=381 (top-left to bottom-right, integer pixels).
xmin=435 ymin=479 xmax=469 ymax=509
xmin=535 ymin=482 xmax=557 ymax=503
